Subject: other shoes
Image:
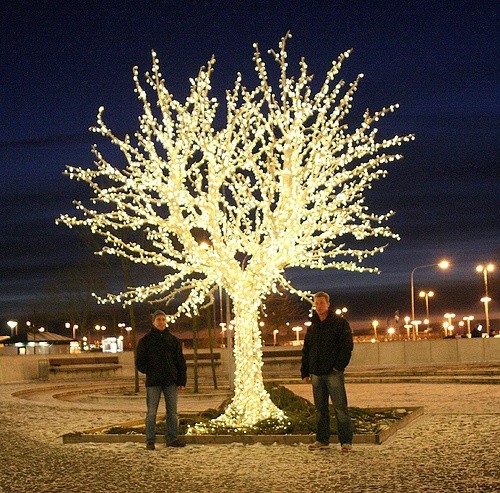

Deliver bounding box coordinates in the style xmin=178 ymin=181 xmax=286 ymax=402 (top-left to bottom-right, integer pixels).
xmin=146 ymin=443 xmax=155 ymax=450
xmin=166 ymin=439 xmax=186 ymax=447
xmin=341 ymin=444 xmax=352 ymax=452
xmin=308 ymin=441 xmax=330 ymax=450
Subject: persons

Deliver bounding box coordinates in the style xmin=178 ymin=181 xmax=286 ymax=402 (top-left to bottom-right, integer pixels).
xmin=135 ymin=310 xmax=187 ymax=450
xmin=300 ymin=291 xmax=354 ymax=451
xmin=489 ymin=329 xmax=496 ymax=338
xmin=471 ymin=324 xmax=482 ymax=338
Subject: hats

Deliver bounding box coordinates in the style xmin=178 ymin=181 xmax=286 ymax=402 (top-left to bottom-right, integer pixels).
xmin=152 ymin=310 xmax=166 ymax=323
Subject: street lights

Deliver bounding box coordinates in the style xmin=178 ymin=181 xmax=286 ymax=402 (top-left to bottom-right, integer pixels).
xmin=411 ymin=260 xmax=450 ymax=341
xmin=273 ymin=291 xmax=491 ymax=344
xmin=476 ymin=264 xmax=495 ymax=337
xmin=7 ymin=319 xmax=132 ymax=355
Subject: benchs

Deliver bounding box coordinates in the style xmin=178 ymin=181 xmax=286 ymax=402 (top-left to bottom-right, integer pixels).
xmin=48 ymin=356 xmax=123 ymax=378
xmin=261 ymin=350 xmax=304 ymax=372
xmin=183 ymin=353 xmax=221 ymax=371
